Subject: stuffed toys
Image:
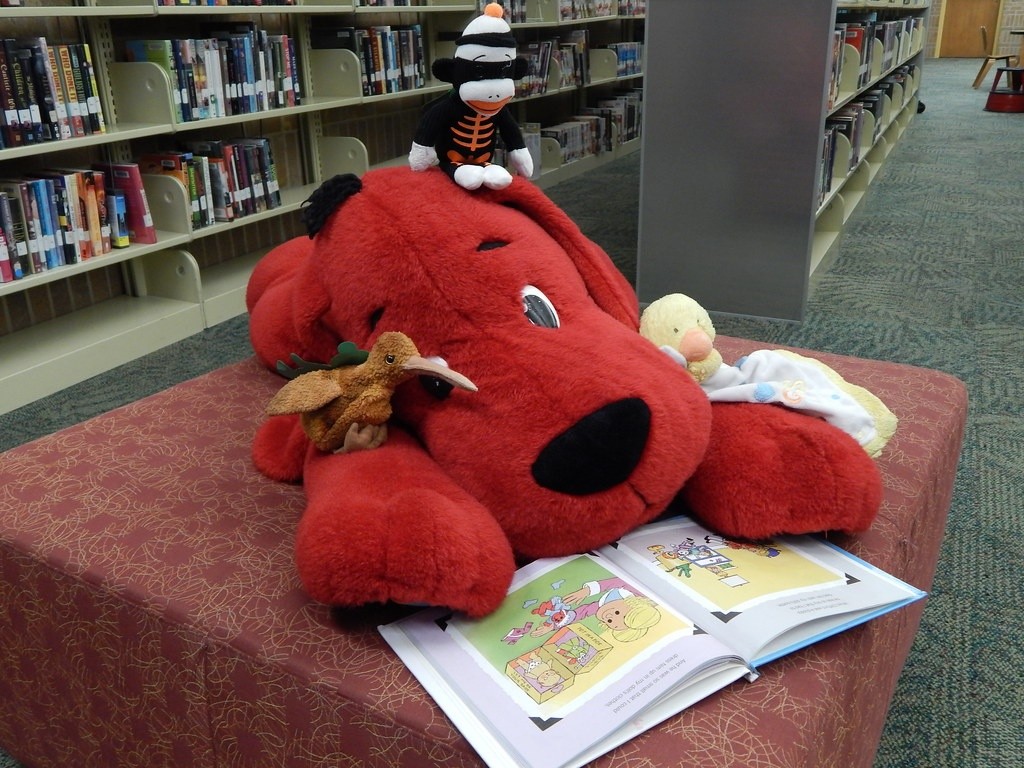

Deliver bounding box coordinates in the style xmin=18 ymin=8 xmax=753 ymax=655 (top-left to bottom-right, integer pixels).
xmin=406 ymin=2 xmax=535 ymax=192
xmin=266 ymin=328 xmax=480 ymax=453
xmin=639 ymin=294 xmax=725 ymax=385
xmin=242 ymin=164 xmax=880 ymax=616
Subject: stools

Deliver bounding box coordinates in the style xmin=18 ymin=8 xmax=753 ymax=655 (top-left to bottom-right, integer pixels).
xmin=983 ymin=67 xmax=1024 ymax=114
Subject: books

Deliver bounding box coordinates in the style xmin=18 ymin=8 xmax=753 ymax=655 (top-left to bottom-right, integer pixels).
xmin=0 ymin=0 xmax=426 ymax=152
xmin=374 ymin=517 xmax=929 ymax=767
xmin=476 ymin=0 xmax=645 ymax=98
xmin=0 ymin=139 xmax=282 ymax=283
xmin=816 ymin=13 xmax=922 ymax=212
xmin=518 ymin=87 xmax=643 ymax=165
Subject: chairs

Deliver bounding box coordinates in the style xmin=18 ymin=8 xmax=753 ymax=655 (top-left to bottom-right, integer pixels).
xmin=971 ymin=26 xmax=1020 ymax=90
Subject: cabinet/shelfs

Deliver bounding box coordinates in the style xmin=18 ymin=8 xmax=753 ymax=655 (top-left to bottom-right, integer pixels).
xmin=0 ymin=0 xmax=479 ymax=416
xmin=479 ymin=0 xmax=646 ymax=190
xmin=634 ymin=0 xmax=933 ymax=323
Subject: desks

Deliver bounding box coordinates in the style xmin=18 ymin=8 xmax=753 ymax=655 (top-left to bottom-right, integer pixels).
xmin=0 ymin=335 xmax=969 ymax=768
xmin=1010 ymin=30 xmax=1024 ymax=67
